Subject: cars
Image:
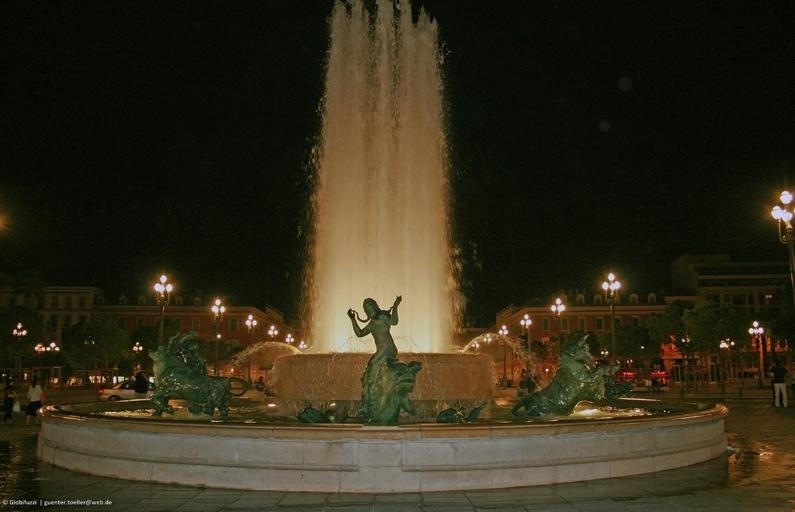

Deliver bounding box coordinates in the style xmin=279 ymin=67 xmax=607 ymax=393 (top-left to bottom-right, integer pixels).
xmin=96 ymin=378 xmax=158 ymax=401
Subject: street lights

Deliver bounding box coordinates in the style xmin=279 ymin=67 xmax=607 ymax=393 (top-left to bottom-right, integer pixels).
xmin=153 ymin=274 xmax=174 ymax=350
xmin=519 ymin=314 xmax=534 ymax=372
xmin=771 ymin=188 xmax=795 ymax=308
xmin=720 ymin=338 xmax=736 ymax=379
xmin=12 ymin=322 xmax=28 ymax=393
xmin=497 ymin=323 xmax=509 ymax=387
xmin=210 ymin=298 xmax=224 ymax=377
xmin=548 ymin=297 xmax=567 ymax=341
xmin=132 ymin=340 xmax=144 ymax=373
xmin=749 ymin=320 xmax=765 ymax=386
xmin=601 ymin=272 xmax=623 ymax=358
xmin=244 ymin=313 xmax=258 ymax=387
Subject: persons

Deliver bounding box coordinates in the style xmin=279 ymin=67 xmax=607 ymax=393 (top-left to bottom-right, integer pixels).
xmin=26 ymin=376 xmax=40 ymax=424
xmin=175 ymin=328 xmax=210 ymax=373
xmin=768 ymin=361 xmax=783 ymax=406
xmin=646 ymin=377 xmax=653 ymax=393
xmin=2 ymin=379 xmax=16 ymax=424
xmin=133 ymin=364 xmax=148 ymax=399
xmin=257 ymin=375 xmax=265 ymax=395
xmin=347 ymin=295 xmax=402 ymax=412
xmin=651 ymin=376 xmax=663 ymax=393
xmin=770 ymin=359 xmax=790 ymax=408
xmin=516 ymin=368 xmax=534 ymax=396
